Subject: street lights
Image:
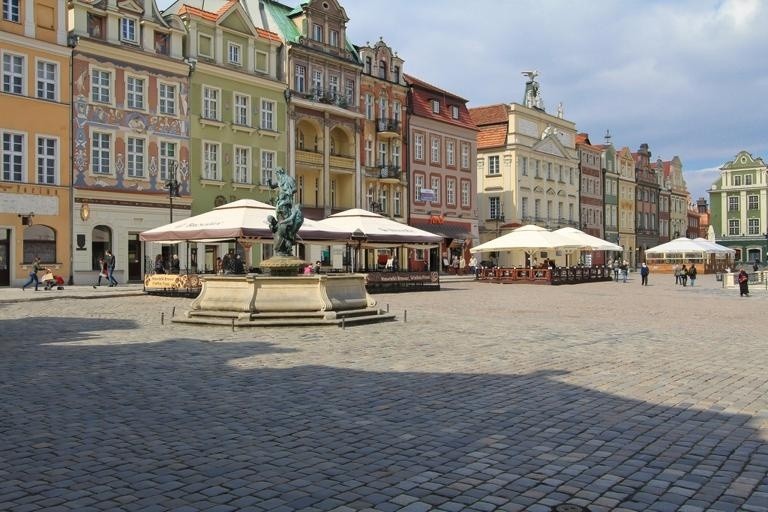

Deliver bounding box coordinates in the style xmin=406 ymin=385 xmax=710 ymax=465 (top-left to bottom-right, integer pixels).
xmin=166 ymin=160 xmax=181 ymax=224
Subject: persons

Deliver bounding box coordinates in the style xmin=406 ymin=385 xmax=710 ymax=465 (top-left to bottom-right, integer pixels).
xmin=450 ymin=255 xmax=459 ymax=276
xmin=680 ymin=265 xmax=689 ymax=286
xmin=608 ymin=258 xmax=612 ymax=276
xmin=303 ymin=262 xmax=313 ymax=274
xmin=737 ymin=267 xmax=749 ymax=297
xmin=469 ymin=256 xmax=477 ymax=276
xmin=689 ymin=263 xmax=697 ymax=287
xmin=269 ymin=164 xmax=297 ymax=220
xmin=543 ymin=259 xmax=549 ymax=269
xmin=620 ymin=264 xmax=627 ymax=283
xmin=105 ymin=249 xmax=118 ymax=287
xmin=313 ymin=260 xmax=321 ymax=274
xmin=22 ymin=256 xmax=49 ymax=291
xmin=170 ymin=254 xmax=180 ymax=274
xmin=533 ymin=257 xmax=538 ymax=277
xmin=613 ymin=260 xmax=619 ymax=282
xmin=457 ymin=255 xmax=466 ymax=276
xmin=216 ymin=257 xmax=223 ymax=271
xmin=673 ymin=262 xmax=683 ymax=285
xmin=547 ymin=258 xmax=557 ymax=270
xmin=155 ymin=253 xmax=167 ymax=274
xmin=280 ymin=202 xmax=304 ymax=242
xmin=640 ymin=261 xmax=650 ymax=285
xmin=95 ymin=255 xmax=114 ymax=286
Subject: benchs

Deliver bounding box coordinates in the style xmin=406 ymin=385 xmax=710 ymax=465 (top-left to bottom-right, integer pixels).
xmin=142 ymin=269 xmax=440 ymax=298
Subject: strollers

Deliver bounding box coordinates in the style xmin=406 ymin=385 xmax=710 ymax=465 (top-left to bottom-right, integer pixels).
xmin=42 ymin=266 xmax=64 ymax=290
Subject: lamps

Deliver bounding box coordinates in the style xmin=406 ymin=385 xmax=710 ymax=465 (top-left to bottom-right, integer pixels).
xmin=17 ymin=211 xmax=34 ymax=227
